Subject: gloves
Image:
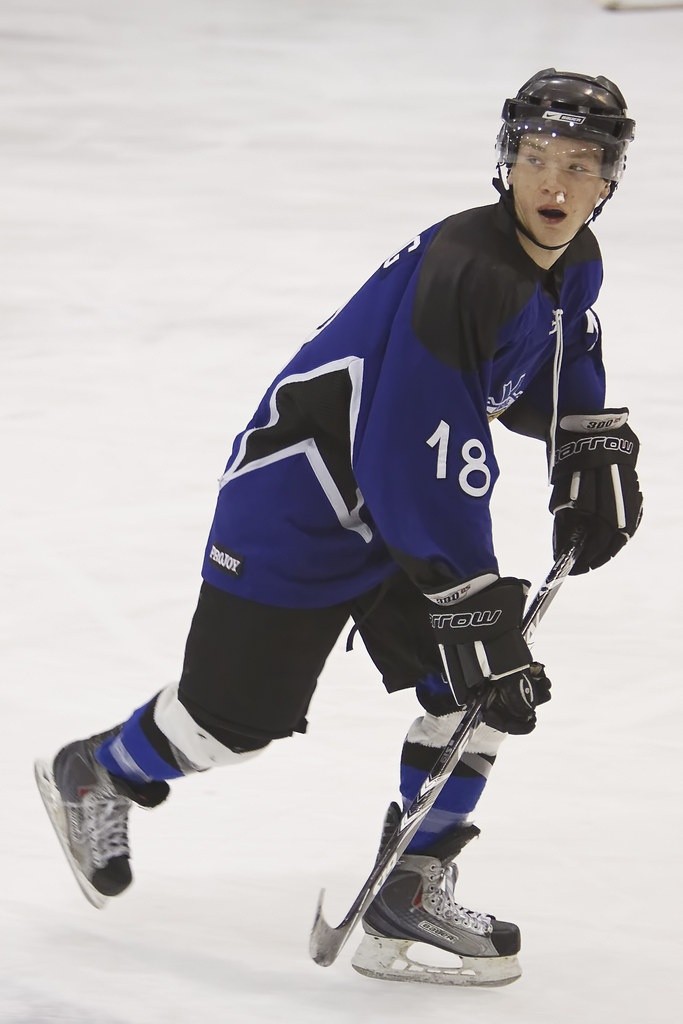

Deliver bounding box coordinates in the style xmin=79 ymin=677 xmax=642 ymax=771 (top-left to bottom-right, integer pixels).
xmin=548 ymin=406 xmax=645 ymax=578
xmin=421 ymin=572 xmax=552 ymax=737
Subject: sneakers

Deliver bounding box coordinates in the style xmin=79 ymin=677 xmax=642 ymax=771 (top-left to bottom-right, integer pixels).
xmin=34 ymin=723 xmax=171 ymax=909
xmin=351 ymin=799 xmax=523 ymax=989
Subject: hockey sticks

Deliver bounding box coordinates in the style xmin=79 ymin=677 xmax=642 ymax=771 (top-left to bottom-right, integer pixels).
xmin=309 ymin=506 xmax=611 ymax=966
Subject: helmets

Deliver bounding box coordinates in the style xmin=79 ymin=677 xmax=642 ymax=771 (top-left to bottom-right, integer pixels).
xmin=498 ymin=68 xmax=636 ymax=165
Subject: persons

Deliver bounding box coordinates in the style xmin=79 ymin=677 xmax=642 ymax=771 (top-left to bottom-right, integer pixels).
xmin=33 ymin=69 xmax=643 ymax=985
